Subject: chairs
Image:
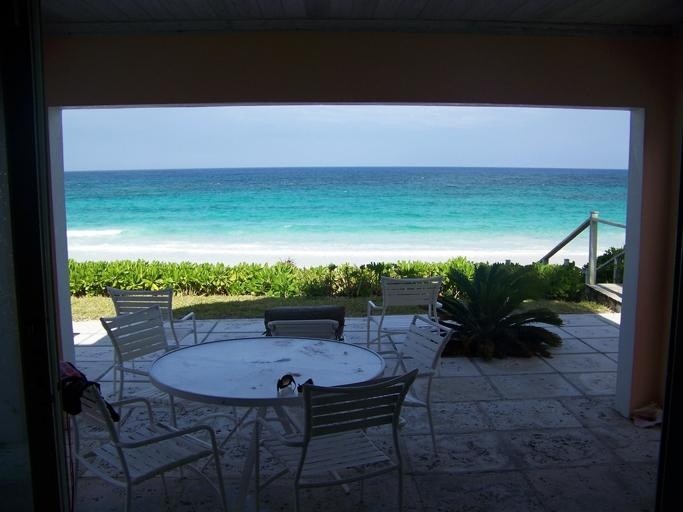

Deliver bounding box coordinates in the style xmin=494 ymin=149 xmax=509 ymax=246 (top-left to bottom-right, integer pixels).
xmin=366 ymin=276 xmax=443 ymax=353
xmin=263 ymin=305 xmax=347 ymax=439
xmin=249 ymin=368 xmax=420 ymax=511
xmin=105 ymin=284 xmax=199 ymax=398
xmin=57 ymin=360 xmax=233 ymax=512
xmin=100 ymin=306 xmax=241 ymax=479
xmin=363 ymin=314 xmax=454 ymax=457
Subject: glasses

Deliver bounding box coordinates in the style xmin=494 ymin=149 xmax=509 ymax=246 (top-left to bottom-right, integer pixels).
xmin=277 ymin=375 xmax=296 ymax=392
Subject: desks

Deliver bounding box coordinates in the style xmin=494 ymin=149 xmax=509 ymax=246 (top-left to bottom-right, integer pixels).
xmin=145 ymin=335 xmax=402 ymax=511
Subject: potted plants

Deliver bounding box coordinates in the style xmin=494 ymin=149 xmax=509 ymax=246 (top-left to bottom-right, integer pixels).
xmin=431 ymin=263 xmax=563 ymax=361
